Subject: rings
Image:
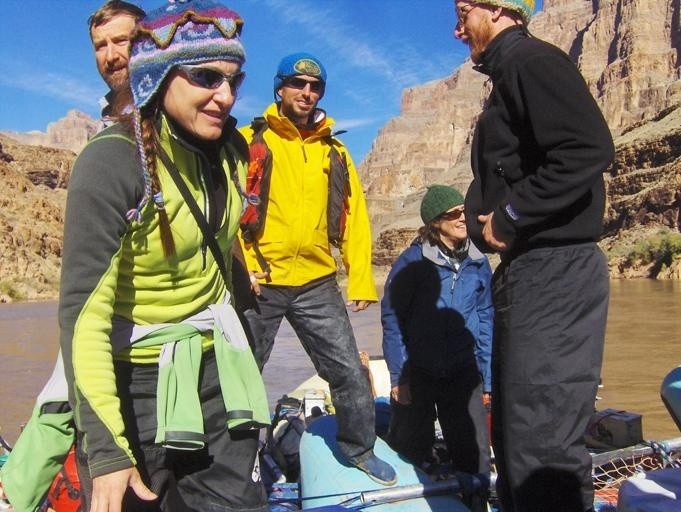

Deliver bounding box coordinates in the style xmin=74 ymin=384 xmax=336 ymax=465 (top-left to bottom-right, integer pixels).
xmin=250 ymin=275 xmax=256 ymax=283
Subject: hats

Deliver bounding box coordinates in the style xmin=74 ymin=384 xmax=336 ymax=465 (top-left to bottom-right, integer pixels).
xmin=466 ymin=0 xmax=536 ymax=24
xmin=121 ymin=1 xmax=261 ymax=108
xmin=421 ymin=185 xmax=465 ymax=224
xmin=273 ymin=53 xmax=326 ymax=103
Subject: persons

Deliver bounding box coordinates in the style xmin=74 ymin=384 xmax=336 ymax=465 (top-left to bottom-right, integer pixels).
xmin=452 ymin=1 xmax=614 ymax=511
xmin=381 ymin=185 xmax=497 ymax=511
xmin=87 ymin=0 xmax=146 ymax=135
xmin=235 ymin=53 xmax=397 ymax=485
xmin=57 ymin=0 xmax=271 ymax=512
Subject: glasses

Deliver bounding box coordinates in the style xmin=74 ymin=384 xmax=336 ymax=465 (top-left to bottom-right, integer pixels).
xmin=438 ymin=208 xmax=466 ymax=222
xmin=276 ymin=73 xmax=323 ymax=92
xmin=455 ymin=5 xmax=478 ymax=24
xmin=174 ymin=63 xmax=247 ymax=92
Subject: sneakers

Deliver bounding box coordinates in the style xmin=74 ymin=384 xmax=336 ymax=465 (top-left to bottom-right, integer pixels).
xmin=344 ymin=452 xmax=396 ymax=486
